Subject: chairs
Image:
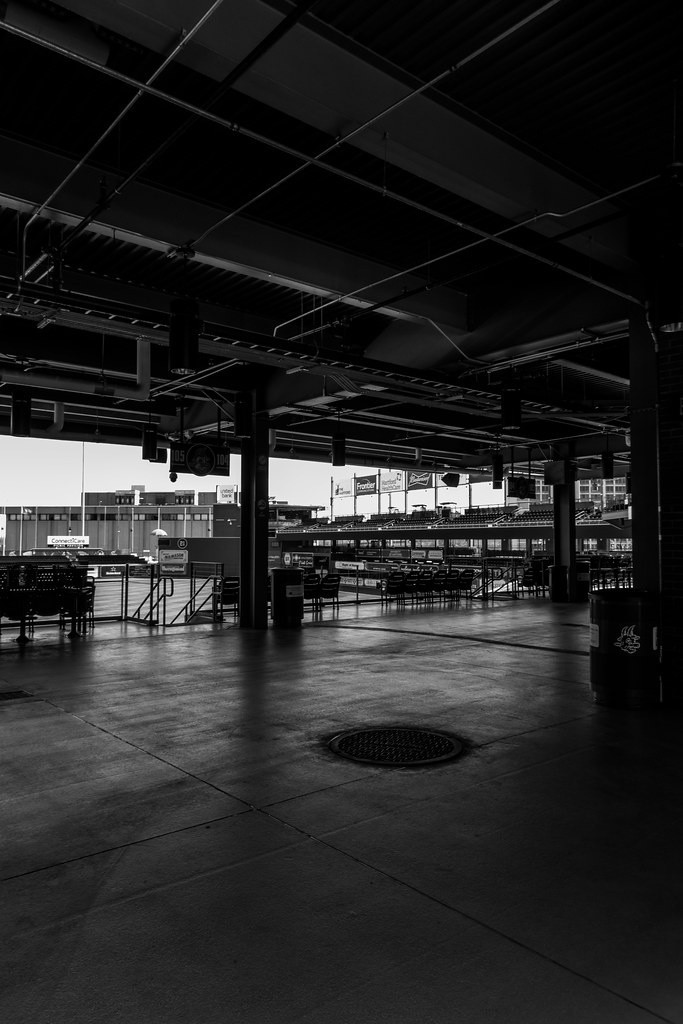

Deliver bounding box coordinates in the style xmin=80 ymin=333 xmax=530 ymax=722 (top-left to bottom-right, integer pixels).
xmin=212 ymin=505 xmax=632 ymax=618
xmin=0 ymin=564 xmax=95 ymax=646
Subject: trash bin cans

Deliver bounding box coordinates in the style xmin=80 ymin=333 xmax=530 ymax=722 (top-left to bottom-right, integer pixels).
xmin=588 ymin=588 xmax=661 ymax=709
xmin=271 ymin=567 xmax=305 ymax=624
xmin=548 ymin=565 xmax=567 ymax=602
xmin=574 ymin=561 xmax=590 ymax=601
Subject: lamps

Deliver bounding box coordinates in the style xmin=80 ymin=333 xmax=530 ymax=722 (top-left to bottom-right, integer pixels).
xmin=142 ymin=395 xmax=157 ymax=459
xmin=441 ymin=473 xmax=460 ymax=487
xmin=500 ymin=396 xmax=521 ymax=430
xmin=168 ymin=248 xmax=202 ymax=375
xmin=330 ymin=412 xmax=345 ymax=466
xmin=10 ymin=393 xmax=30 ymax=437
xmin=491 ymin=432 xmax=504 ymax=482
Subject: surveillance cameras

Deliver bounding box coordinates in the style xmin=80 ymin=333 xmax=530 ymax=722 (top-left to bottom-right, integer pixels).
xmin=170 ymin=474 xmax=177 ymax=482
